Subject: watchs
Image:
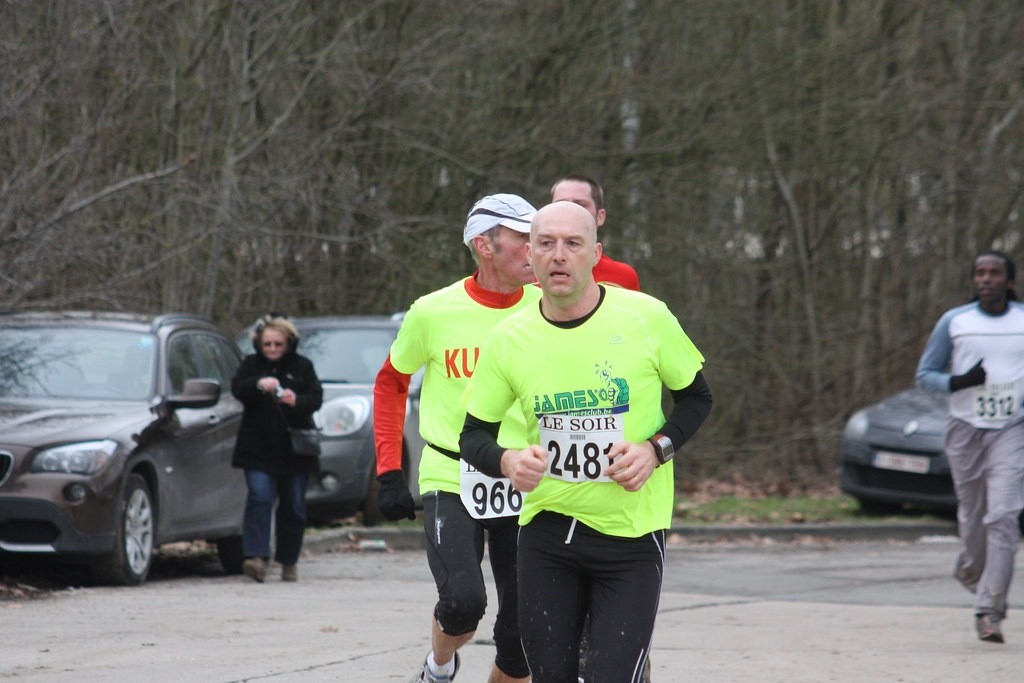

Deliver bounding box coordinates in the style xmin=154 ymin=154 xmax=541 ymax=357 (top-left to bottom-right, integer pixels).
xmin=646 ymin=433 xmax=675 ymax=468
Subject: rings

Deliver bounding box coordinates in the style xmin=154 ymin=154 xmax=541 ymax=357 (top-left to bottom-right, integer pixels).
xmin=634 ymin=476 xmax=640 ymax=483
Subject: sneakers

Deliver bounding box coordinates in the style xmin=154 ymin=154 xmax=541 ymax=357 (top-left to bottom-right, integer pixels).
xmin=978 ymin=614 xmax=1003 ymax=643
xmin=411 ymin=648 xmax=461 ymax=683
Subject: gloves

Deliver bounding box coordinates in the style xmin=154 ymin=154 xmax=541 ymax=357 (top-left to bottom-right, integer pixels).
xmin=949 ymin=359 xmax=986 ymax=392
xmin=377 ymin=469 xmax=416 ymax=520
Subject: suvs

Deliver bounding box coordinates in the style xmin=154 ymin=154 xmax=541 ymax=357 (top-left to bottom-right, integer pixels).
xmin=0 ymin=309 xmax=245 ymax=585
xmin=229 ymin=311 xmax=430 ymax=527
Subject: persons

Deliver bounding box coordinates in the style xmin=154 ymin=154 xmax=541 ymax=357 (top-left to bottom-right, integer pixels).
xmin=231 ymin=318 xmax=323 ymax=583
xmin=373 ymin=194 xmax=542 ymax=683
xmin=459 ymin=201 xmax=712 ymax=683
xmin=915 ymin=249 xmax=1024 ymax=642
xmin=532 ymin=174 xmax=639 ymax=291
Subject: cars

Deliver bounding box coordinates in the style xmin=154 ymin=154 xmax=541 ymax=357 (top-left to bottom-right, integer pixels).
xmin=836 ymin=389 xmax=958 ymax=520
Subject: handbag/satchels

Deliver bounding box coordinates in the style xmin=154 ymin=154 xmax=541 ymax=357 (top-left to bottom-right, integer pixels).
xmin=290 ymin=429 xmax=321 ymax=456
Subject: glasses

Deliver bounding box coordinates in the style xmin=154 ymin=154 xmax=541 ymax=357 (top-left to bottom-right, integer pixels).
xmin=263 ymin=341 xmax=282 ymax=346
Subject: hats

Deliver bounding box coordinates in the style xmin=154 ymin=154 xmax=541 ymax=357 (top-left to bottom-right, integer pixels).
xmin=464 ymin=194 xmax=537 ymax=246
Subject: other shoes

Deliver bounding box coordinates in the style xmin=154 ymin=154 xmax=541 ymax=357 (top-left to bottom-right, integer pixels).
xmin=282 ymin=565 xmax=297 ymax=580
xmin=244 ymin=557 xmax=265 ymax=582
